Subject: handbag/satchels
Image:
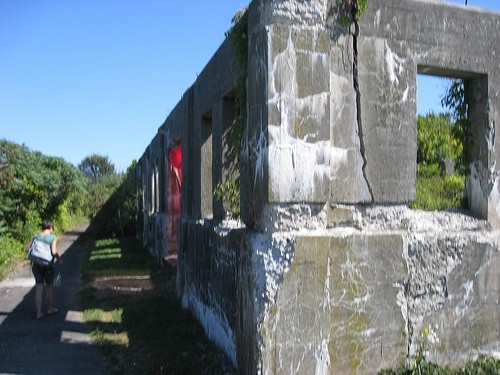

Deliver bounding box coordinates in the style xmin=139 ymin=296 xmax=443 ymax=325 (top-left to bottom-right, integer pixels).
xmin=26 ymin=234 xmax=55 ymax=268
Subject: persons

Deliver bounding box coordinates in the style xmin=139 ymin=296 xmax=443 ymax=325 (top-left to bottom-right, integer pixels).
xmin=26 ymin=219 xmax=62 ymax=320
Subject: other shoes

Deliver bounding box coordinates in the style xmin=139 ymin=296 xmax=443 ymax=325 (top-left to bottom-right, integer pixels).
xmin=35 ymin=308 xmax=58 ymax=320
